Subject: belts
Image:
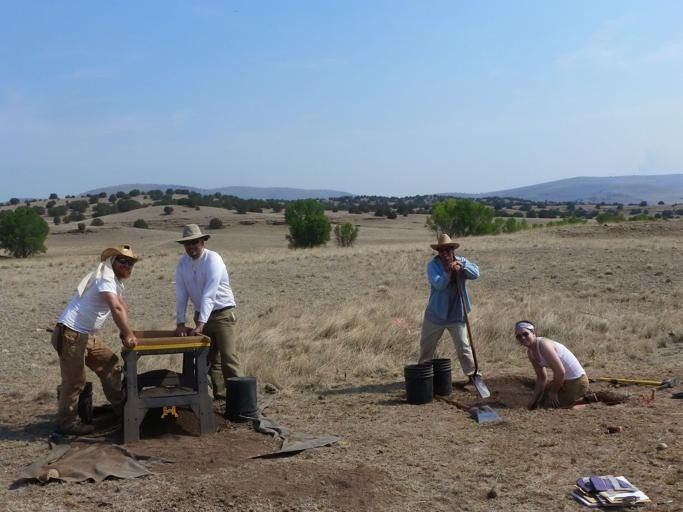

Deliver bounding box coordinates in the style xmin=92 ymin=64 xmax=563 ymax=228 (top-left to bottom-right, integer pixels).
xmin=195 ymin=306 xmax=235 ymax=315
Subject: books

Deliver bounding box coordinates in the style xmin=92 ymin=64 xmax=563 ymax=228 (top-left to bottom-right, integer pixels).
xmin=569 ymin=474 xmax=651 ymax=508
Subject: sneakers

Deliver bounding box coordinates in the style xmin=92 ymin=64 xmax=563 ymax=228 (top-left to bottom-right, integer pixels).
xmin=57 ymin=423 xmax=95 ymax=435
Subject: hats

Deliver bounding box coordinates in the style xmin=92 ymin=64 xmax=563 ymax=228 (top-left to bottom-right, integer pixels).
xmin=101 ymin=244 xmax=143 ymax=262
xmin=430 ymin=233 xmax=460 ymax=251
xmin=176 ymin=223 xmax=211 ymax=244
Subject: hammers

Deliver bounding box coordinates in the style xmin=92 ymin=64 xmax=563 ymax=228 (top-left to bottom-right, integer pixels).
xmin=596 ymin=377 xmax=679 ymax=391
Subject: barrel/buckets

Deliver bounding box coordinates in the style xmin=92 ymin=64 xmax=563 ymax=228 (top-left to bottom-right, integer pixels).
xmin=56 ymin=383 xmax=94 ymax=418
xmin=426 ymin=358 xmax=452 ymax=396
xmin=225 ymin=377 xmax=260 ymax=428
xmin=404 ymin=364 xmax=434 ymax=403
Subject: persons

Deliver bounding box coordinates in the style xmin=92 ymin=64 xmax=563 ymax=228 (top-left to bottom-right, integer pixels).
xmin=173 ymin=224 xmax=244 ymax=411
xmin=416 ymin=232 xmax=483 ymax=392
xmin=515 ymin=319 xmax=602 ymax=412
xmin=50 ymin=243 xmax=146 ymax=435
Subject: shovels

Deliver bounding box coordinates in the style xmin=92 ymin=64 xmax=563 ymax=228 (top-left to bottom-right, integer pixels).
xmin=455 ymin=272 xmax=491 ymax=399
xmin=434 ymin=395 xmax=502 ymax=423
